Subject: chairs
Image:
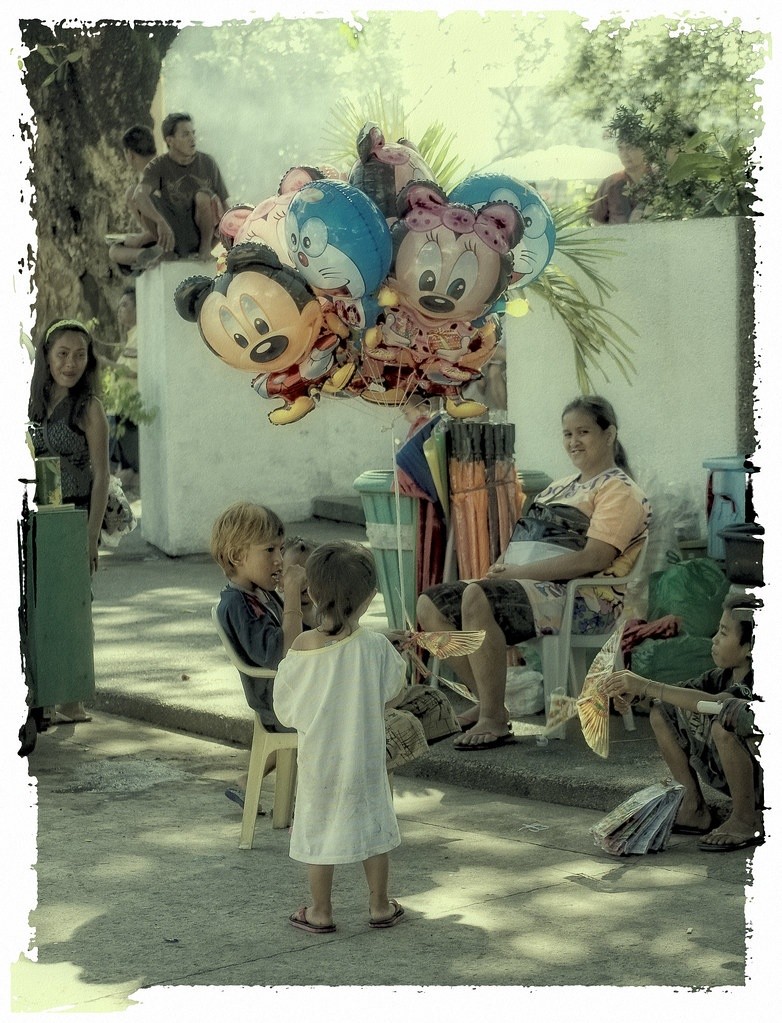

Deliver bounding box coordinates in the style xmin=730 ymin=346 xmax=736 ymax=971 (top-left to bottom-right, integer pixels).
xmin=430 ymin=532 xmax=649 ymax=741
xmin=211 ymin=602 xmax=395 ymax=851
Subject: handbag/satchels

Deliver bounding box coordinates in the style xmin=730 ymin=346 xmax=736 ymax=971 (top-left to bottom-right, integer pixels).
xmin=100 ymin=474 xmax=133 ymax=535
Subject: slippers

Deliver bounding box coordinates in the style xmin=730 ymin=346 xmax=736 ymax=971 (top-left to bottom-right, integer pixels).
xmin=453 ymin=712 xmax=512 ymax=730
xmin=368 ymin=898 xmax=405 ymax=928
xmin=51 ymin=712 xmax=92 ymax=725
xmin=670 ymin=821 xmax=711 ymax=836
xmin=454 ymin=727 xmax=518 ymax=750
xmin=225 ymin=788 xmax=266 ymax=816
xmin=696 ymin=823 xmax=764 ymax=851
xmin=288 ymin=907 xmax=336 ymax=933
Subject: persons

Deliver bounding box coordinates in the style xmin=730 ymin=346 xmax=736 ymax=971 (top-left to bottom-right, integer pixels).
xmin=586 ymin=123 xmax=722 ymax=225
xmin=211 ymin=501 xmax=462 ymax=816
xmin=123 ymin=113 xmax=230 ymax=261
xmin=273 ymin=539 xmax=406 ymax=934
xmin=28 ymin=320 xmax=111 ymax=726
xmin=416 ymin=396 xmax=650 ymax=753
xmin=603 ymin=585 xmax=765 ymax=850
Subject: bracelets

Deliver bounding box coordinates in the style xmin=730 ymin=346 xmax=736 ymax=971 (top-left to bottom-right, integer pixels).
xmin=644 ymin=680 xmax=664 ymax=702
xmin=281 ymin=608 xmax=304 ymax=618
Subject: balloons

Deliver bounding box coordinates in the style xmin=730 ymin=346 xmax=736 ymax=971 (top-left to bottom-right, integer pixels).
xmin=173 ymin=126 xmax=556 ymax=425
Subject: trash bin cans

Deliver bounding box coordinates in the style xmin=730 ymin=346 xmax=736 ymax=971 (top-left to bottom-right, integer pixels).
xmin=515 ymin=469 xmax=554 ymax=520
xmin=352 ymin=469 xmax=417 ymax=632
xmin=702 ymin=456 xmax=745 ymax=561
